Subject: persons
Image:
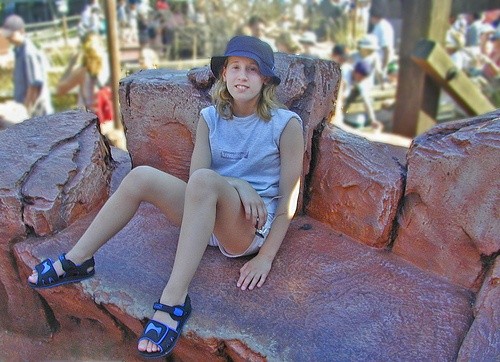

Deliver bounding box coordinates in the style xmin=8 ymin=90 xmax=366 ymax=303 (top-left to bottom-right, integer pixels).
xmin=0 ymin=0 xmax=500 ymax=134
xmin=27 ymin=35 xmax=304 ymax=359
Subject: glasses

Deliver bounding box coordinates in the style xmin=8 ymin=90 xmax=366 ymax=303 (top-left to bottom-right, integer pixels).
xmin=254 ymin=196 xmax=284 ymax=239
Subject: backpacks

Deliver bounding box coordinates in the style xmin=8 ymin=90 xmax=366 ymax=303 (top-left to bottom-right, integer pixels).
xmin=98 ymin=86 xmax=114 ymax=123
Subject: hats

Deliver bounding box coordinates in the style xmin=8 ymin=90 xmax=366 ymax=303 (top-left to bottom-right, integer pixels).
xmin=356 ymin=35 xmax=381 ymax=50
xmin=0 ymin=15 xmax=24 ymax=36
xmin=210 ymin=35 xmax=281 ymax=86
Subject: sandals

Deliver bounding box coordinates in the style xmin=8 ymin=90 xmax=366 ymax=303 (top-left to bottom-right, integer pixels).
xmin=28 ymin=253 xmax=95 ymax=288
xmin=137 ymin=293 xmax=192 ymax=358
